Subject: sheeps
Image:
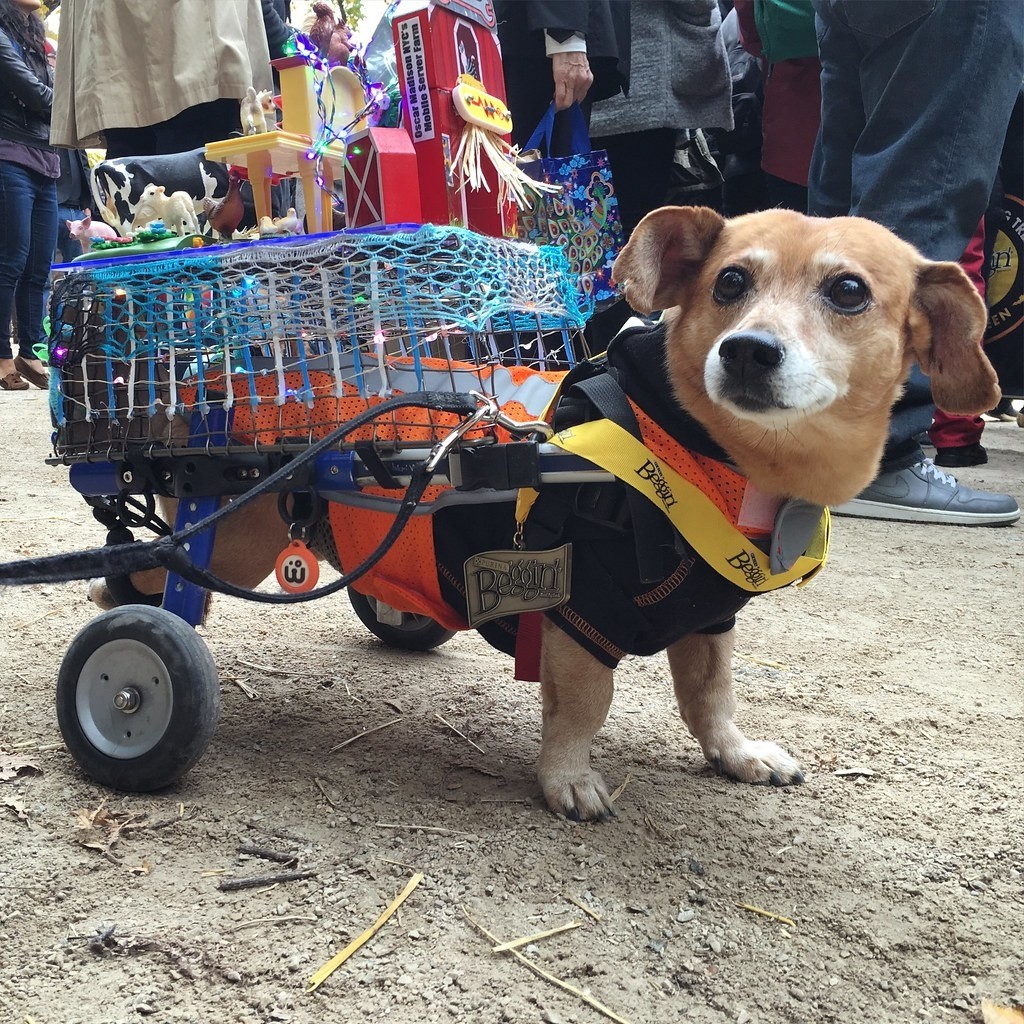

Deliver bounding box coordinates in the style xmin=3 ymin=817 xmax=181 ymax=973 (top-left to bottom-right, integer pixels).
xmin=238 ymin=86 xmax=277 ymax=135
xmin=141 ymin=182 xmax=200 ymax=236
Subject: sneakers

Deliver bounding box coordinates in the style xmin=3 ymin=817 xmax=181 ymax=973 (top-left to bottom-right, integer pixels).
xmin=827 ymin=457 xmax=1021 ymax=525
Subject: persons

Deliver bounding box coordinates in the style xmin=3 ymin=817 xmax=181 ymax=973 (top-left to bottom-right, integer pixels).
xmin=1 ymin=0 xmax=1024 ymax=526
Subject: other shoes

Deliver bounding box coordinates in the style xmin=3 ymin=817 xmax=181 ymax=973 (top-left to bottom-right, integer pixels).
xmin=14 ymin=355 xmax=50 ymax=390
xmin=0 ymin=372 xmax=29 ymax=390
xmin=933 ymin=444 xmax=988 ymax=468
xmin=985 ymin=403 xmax=1019 ymax=421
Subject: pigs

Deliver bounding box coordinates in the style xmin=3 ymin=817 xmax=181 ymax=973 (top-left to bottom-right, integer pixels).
xmin=65 ymin=216 xmax=116 ymax=255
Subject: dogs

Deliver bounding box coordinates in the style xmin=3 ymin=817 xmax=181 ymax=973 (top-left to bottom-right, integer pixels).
xmin=89 ymin=204 xmax=1001 ymax=822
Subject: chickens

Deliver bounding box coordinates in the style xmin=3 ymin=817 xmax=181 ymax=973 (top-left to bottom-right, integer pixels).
xmin=257 ymin=207 xmax=302 ymax=235
xmin=202 ymin=170 xmax=244 ymax=240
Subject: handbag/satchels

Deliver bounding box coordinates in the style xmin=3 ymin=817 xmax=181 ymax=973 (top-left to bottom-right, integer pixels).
xmin=513 ymin=97 xmax=626 ymax=322
